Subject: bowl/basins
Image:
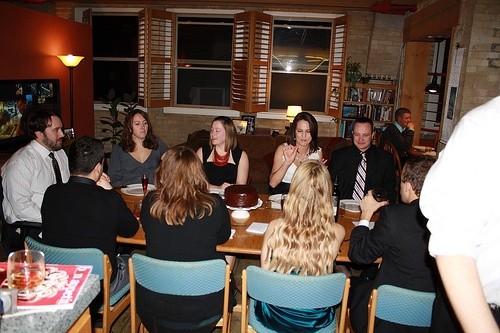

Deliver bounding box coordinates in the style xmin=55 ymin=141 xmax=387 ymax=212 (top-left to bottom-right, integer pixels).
xmin=231 ymin=209 xmax=250 ymax=223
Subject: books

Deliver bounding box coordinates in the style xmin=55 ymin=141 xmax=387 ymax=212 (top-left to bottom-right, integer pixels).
xmin=1 ymin=261 xmax=94 ymax=312
xmin=359 ymin=90 xmax=395 ymax=120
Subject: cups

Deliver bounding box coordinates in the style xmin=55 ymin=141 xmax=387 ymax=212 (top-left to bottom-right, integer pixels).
xmin=339 ymin=204 xmax=345 ymax=216
xmin=372 ymin=188 xmax=387 ymax=215
xmin=8 ymin=250 xmax=45 ymax=300
xmin=281 ymin=194 xmax=288 ymax=211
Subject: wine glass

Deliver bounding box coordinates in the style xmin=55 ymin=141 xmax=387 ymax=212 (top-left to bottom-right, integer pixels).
xmin=140 ymin=173 xmax=148 ymax=203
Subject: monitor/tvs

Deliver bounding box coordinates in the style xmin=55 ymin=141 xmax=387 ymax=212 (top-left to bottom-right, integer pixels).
xmin=1 ymin=78 xmax=61 ymax=153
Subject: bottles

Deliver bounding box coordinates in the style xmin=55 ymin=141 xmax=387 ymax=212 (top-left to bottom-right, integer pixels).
xmin=331 ymin=175 xmax=340 ymax=223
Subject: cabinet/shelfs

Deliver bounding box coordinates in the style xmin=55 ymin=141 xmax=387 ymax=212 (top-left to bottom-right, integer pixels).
xmin=342 ymin=74 xmax=398 ymax=151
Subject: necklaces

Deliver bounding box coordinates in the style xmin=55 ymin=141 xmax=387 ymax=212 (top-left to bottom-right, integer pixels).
xmin=212 ymin=146 xmax=230 ymax=168
xmin=295 ymin=156 xmax=307 ymax=164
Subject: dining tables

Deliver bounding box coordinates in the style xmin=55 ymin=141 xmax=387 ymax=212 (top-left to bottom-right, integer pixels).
xmin=113 ymin=183 xmax=383 ymax=265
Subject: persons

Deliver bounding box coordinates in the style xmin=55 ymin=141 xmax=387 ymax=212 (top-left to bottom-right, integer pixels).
xmin=137 ymin=145 xmax=240 ymax=321
xmin=13 ymin=94 xmax=36 ymax=138
xmin=1 ymin=106 xmax=71 ymax=252
xmin=268 ymin=111 xmax=329 ymax=198
xmin=417 ymin=94 xmax=500 ymax=333
xmin=326 ymin=117 xmax=399 ymax=208
xmin=109 ymin=109 xmax=169 ymax=190
xmin=346 ymin=155 xmax=451 ymax=333
xmin=349 ymin=90 xmax=361 ymax=102
xmin=253 ymin=158 xmax=346 ymax=333
xmin=40 ymin=135 xmax=130 ymax=298
xmin=379 ymin=108 xmax=414 ymax=166
xmin=195 ymin=115 xmax=249 ymax=273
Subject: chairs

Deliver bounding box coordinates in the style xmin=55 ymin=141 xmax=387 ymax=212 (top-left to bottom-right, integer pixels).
xmin=25 ymin=238 xmax=130 ymax=333
xmin=368 ymin=285 xmax=435 ymax=333
xmin=241 ymin=266 xmax=352 ymax=333
xmin=383 ymin=139 xmax=403 ymax=179
xmin=127 ymin=254 xmax=234 ymax=332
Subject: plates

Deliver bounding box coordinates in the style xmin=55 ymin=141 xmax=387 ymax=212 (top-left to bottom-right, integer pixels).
xmin=413 ymin=146 xmax=435 ymax=151
xmin=226 ymin=198 xmax=263 ymax=210
xmin=269 ymin=194 xmax=283 ymax=206
xmin=120 ymin=183 xmax=157 ymax=196
xmin=339 ymin=199 xmax=363 ymax=213
xmin=207 ymin=188 xmax=226 ymax=200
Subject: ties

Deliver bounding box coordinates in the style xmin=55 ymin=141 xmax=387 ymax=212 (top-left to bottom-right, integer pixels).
xmin=351 ymin=153 xmax=368 ymax=201
xmin=48 ymin=152 xmax=62 ymax=184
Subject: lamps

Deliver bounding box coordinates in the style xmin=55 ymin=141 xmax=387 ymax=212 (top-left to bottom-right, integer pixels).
xmin=286 ymin=105 xmax=302 ymax=131
xmin=424 ymin=41 xmax=443 ymax=95
xmin=58 ymin=54 xmax=84 ymax=138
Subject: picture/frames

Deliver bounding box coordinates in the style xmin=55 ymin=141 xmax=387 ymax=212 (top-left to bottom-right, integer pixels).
xmin=62 ymin=126 xmax=76 ymax=142
xmin=232 ymin=115 xmax=257 ymax=136
xmin=1 ymin=78 xmax=61 ymax=152
